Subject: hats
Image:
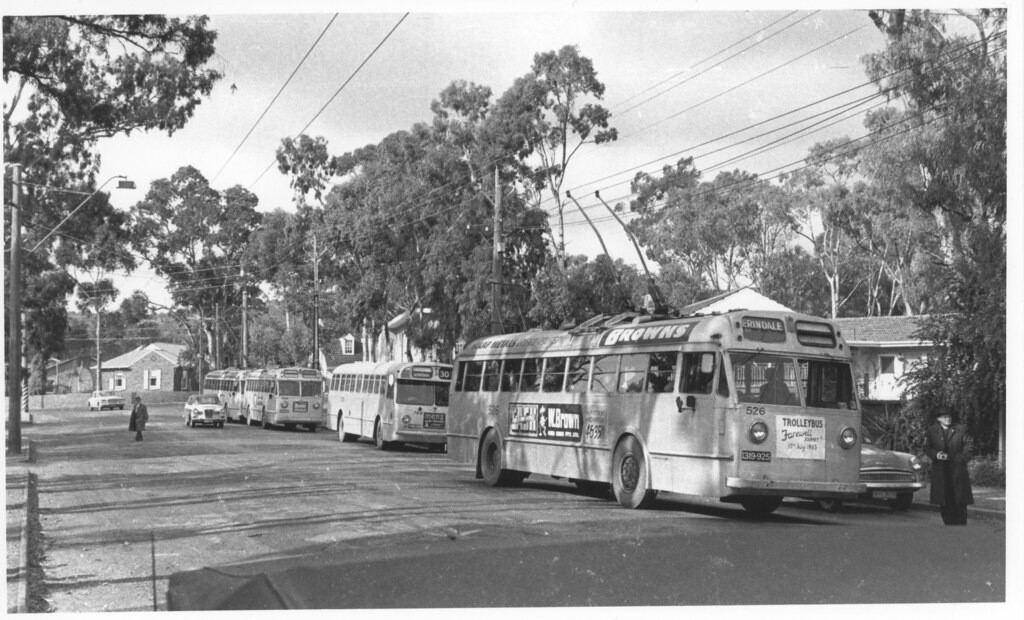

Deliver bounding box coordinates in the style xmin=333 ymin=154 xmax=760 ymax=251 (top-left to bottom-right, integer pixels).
xmin=933 ymin=407 xmax=953 ymax=416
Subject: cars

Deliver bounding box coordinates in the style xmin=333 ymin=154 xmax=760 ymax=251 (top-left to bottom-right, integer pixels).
xmin=183 ymin=394 xmax=227 ymax=428
xmin=815 ymin=441 xmax=928 ymax=512
xmin=88 ymin=390 xmax=124 ymax=410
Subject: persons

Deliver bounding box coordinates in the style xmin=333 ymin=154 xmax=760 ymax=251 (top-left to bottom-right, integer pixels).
xmin=922 ymin=404 xmax=975 ymax=526
xmin=603 ymin=364 xmax=674 ymax=393
xmin=129 ymin=396 xmax=149 ymax=441
xmin=760 ymin=368 xmax=792 ymax=405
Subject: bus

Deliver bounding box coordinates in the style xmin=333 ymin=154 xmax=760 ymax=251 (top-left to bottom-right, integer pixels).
xmin=327 ymin=361 xmax=453 ymax=450
xmin=202 ymin=367 xmax=245 ymax=423
xmin=245 ymin=366 xmax=323 ymax=432
xmin=448 ymin=309 xmax=868 ymax=516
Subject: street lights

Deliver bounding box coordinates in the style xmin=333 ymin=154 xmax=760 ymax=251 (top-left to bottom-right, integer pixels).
xmin=7 ymin=162 xmax=137 ymax=455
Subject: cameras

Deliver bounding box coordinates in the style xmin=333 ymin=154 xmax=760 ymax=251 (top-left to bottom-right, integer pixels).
xmin=939 ymin=453 xmax=950 ymax=460
